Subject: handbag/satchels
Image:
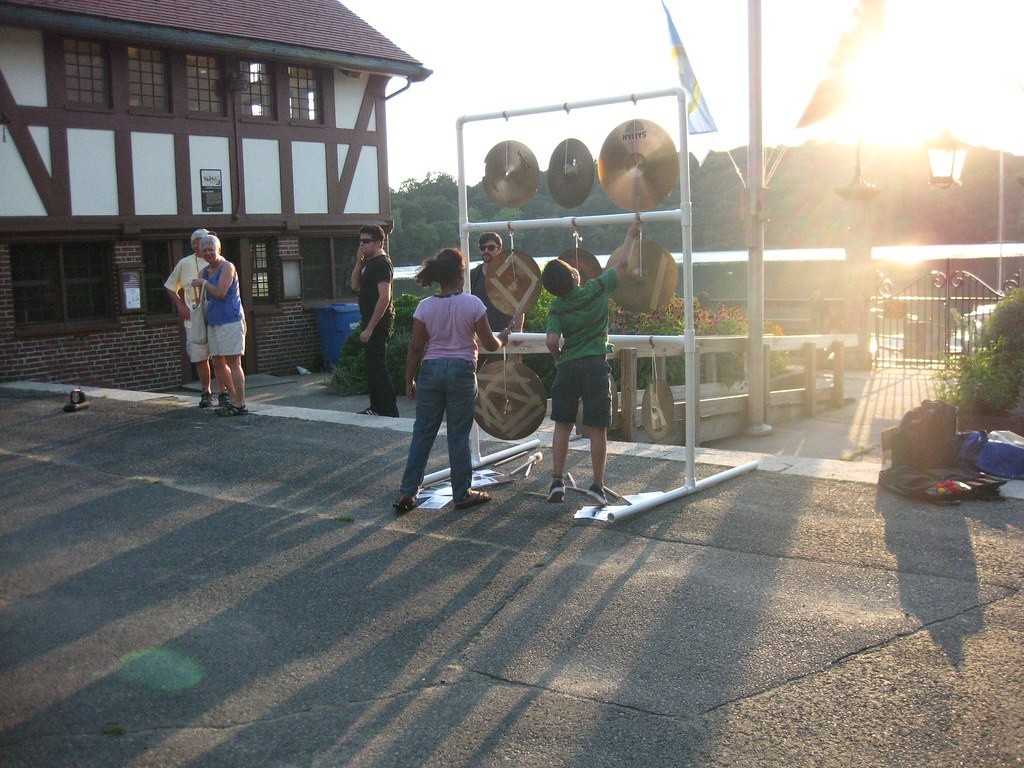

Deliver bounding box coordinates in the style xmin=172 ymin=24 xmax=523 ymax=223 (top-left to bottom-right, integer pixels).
xmin=189 ymin=280 xmax=208 ymax=345
xmin=891 ymin=400 xmax=957 ymax=468
xmin=952 ymin=427 xmax=1024 ymax=479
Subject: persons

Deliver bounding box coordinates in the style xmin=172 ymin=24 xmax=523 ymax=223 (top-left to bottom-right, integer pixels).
xmin=351 ymin=224 xmax=399 ymax=417
xmin=469 ymin=232 xmax=524 ymax=374
xmin=393 ymin=248 xmax=512 ymax=510
xmin=191 ymin=235 xmax=249 ymax=416
xmin=164 ymin=229 xmax=232 ymax=407
xmin=541 ymin=220 xmax=643 ymax=505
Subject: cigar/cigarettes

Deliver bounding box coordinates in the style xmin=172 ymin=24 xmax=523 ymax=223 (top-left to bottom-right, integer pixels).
xmin=360 ymin=248 xmax=363 ymax=250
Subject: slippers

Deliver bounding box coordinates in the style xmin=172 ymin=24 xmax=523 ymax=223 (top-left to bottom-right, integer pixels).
xmin=454 ymin=490 xmax=492 ymax=509
xmin=392 ymin=495 xmax=418 ymax=510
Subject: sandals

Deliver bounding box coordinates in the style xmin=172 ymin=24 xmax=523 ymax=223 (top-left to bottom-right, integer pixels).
xmin=214 ymin=402 xmax=249 ymax=417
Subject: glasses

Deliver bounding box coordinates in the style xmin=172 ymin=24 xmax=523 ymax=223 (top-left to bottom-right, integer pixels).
xmin=358 ymin=238 xmax=374 ymax=244
xmin=477 ymin=244 xmax=500 ymax=250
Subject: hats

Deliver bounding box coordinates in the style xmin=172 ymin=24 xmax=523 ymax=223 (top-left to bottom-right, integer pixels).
xmin=191 ymin=228 xmax=217 ymax=241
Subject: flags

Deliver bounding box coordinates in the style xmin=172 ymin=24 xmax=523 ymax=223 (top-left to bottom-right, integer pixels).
xmin=663 ymin=5 xmax=717 ymax=134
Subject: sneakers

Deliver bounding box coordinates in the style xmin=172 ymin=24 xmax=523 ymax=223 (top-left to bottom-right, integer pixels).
xmin=218 ymin=392 xmax=231 ymax=410
xmin=199 ymin=390 xmax=213 ymax=407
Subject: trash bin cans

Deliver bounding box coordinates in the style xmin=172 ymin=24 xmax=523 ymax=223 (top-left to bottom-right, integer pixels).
xmin=309 ymin=302 xmax=361 ymax=374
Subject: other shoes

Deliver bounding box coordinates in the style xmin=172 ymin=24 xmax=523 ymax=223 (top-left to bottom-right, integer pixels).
xmin=353 ymin=407 xmax=400 ymax=417
xmin=586 ymin=484 xmax=608 ymax=506
xmin=546 ymin=482 xmax=566 ymax=502
